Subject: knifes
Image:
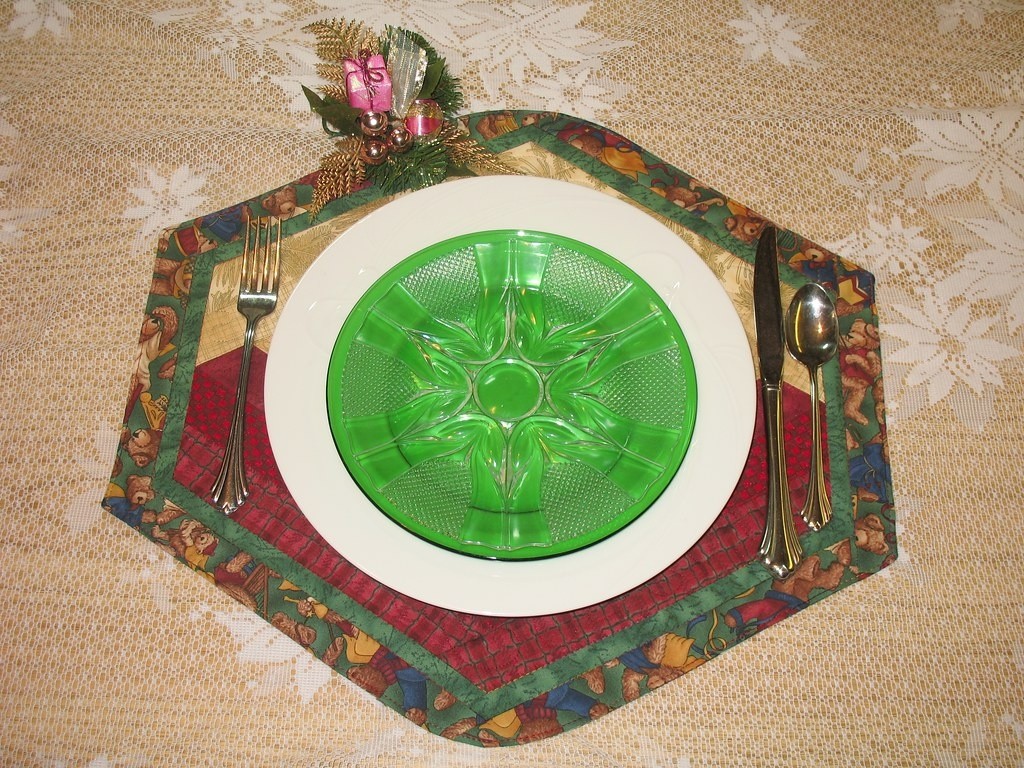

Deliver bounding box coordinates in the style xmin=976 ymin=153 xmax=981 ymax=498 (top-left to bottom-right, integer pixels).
xmin=753 ymin=227 xmax=803 ymax=581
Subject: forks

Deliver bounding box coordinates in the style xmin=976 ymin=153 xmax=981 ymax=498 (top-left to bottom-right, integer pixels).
xmin=211 ymin=216 xmax=282 ymax=516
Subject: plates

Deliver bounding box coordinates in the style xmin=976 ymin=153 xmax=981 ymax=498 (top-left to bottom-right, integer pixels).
xmin=264 ymin=175 xmax=756 ymax=616
xmin=325 ymin=230 xmax=698 ymax=562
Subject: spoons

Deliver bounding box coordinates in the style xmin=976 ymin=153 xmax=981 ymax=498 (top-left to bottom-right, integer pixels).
xmin=785 ymin=284 xmax=838 ymax=530
